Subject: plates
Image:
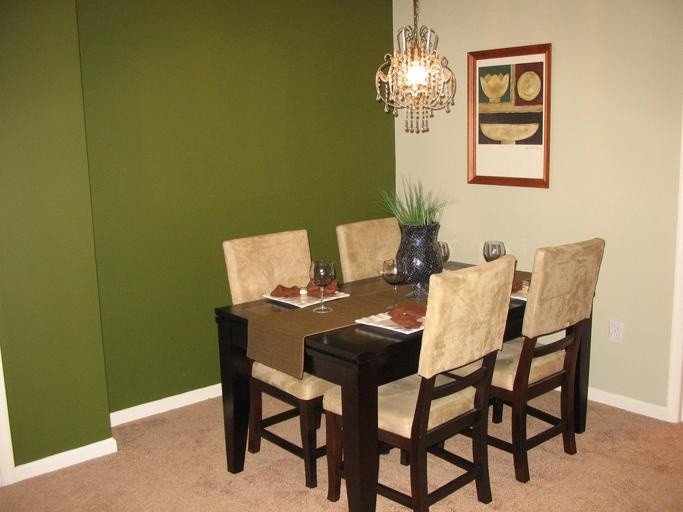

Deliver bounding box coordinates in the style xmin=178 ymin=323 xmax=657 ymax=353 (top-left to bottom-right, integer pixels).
xmin=355 ymin=310 xmax=425 ymax=335
xmin=262 ymin=286 xmax=351 ymax=308
xmin=517 ymin=71 xmax=541 ymax=101
xmin=510 ymin=280 xmax=531 ymax=302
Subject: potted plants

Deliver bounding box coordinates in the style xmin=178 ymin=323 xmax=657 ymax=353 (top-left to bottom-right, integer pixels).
xmin=374 ymin=172 xmax=456 ymax=301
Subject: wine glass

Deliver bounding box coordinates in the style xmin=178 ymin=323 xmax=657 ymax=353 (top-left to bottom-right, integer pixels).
xmin=382 ymin=259 xmax=406 ymax=310
xmin=438 ymin=241 xmax=450 ymax=269
xmin=309 ymin=261 xmax=336 ymax=313
xmin=483 ymin=241 xmax=506 ymax=263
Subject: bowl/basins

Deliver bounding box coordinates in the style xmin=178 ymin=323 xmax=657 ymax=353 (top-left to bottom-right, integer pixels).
xmin=479 ymin=73 xmax=510 ymax=103
xmin=480 ymin=122 xmax=540 ymax=144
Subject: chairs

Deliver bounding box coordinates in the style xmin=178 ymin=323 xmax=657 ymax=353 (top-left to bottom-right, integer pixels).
xmin=441 ymin=237 xmax=606 ymax=482
xmin=335 ymin=216 xmax=402 ymax=284
xmin=221 ymin=227 xmax=338 ymax=488
xmin=322 ymin=254 xmax=517 ymax=512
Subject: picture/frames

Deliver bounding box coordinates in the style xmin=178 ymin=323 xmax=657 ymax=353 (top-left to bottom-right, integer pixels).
xmin=467 ymin=42 xmax=552 ymax=188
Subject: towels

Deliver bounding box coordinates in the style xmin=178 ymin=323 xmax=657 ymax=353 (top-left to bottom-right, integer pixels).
xmin=269 ymin=277 xmax=339 ymax=299
xmin=388 ymin=305 xmax=427 ymax=329
xmin=510 ymin=278 xmax=523 ymax=293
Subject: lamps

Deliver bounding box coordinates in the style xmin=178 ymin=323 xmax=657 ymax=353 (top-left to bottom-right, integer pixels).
xmin=374 ymin=2 xmax=457 ymax=133
